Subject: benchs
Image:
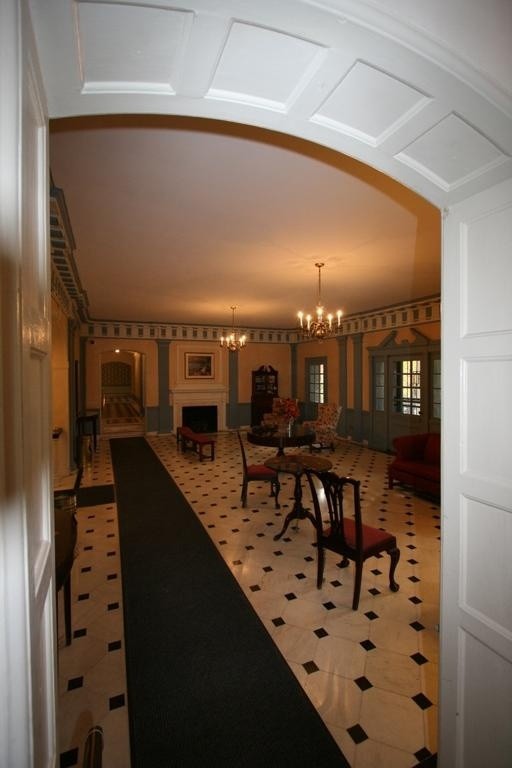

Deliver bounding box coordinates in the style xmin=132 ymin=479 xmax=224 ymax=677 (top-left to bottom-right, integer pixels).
xmin=176 ymin=426 xmax=217 ymax=462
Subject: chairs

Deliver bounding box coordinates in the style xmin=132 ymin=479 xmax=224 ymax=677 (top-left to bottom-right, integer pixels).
xmin=302 ymin=403 xmax=343 ymax=454
xmin=236 ymin=429 xmax=283 ymax=509
xmin=304 ymin=466 xmax=401 ymax=610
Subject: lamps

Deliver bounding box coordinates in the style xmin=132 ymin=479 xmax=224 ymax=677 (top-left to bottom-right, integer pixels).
xmin=218 ymin=305 xmax=249 ymax=352
xmin=295 ymin=263 xmax=343 ymax=344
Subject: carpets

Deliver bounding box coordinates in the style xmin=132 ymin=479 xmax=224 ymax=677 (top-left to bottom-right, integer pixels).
xmin=110 ymin=437 xmax=350 ymax=767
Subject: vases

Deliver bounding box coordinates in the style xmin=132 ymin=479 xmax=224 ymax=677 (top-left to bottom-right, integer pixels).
xmin=277 ymin=416 xmax=292 ymax=433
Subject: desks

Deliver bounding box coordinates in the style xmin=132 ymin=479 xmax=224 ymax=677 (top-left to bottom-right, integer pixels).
xmin=246 ymin=424 xmax=317 ymax=457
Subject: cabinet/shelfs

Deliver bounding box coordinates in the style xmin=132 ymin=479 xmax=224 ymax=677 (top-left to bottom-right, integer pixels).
xmin=251 ymin=364 xmax=279 ymax=428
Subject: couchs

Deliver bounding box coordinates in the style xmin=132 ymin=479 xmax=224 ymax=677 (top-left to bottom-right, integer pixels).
xmin=386 ymin=432 xmax=440 ymax=504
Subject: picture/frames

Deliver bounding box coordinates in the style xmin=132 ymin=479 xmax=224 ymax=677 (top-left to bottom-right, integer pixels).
xmin=184 ymin=352 xmax=215 ymax=379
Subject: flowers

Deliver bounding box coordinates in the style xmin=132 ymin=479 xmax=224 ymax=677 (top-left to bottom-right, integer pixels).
xmin=269 ymin=396 xmax=301 ymax=425
